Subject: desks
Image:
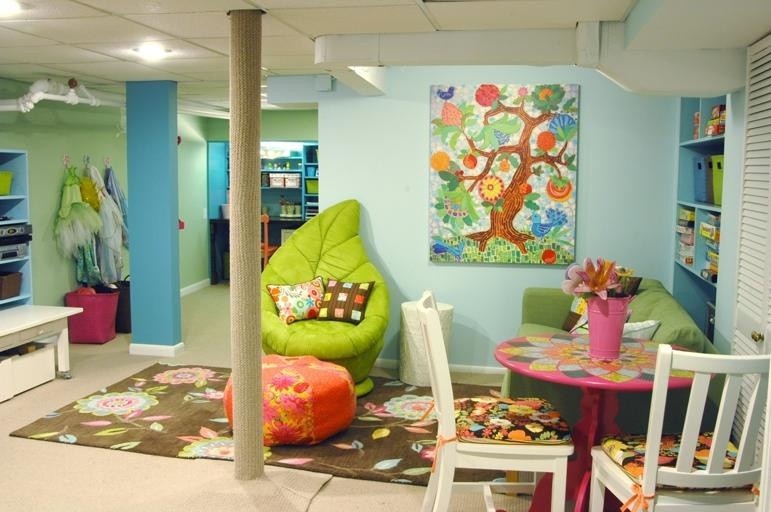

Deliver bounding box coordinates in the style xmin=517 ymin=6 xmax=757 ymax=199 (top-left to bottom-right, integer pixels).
xmin=0 ymin=305 xmax=86 ymax=373
xmin=401 ymin=299 xmax=455 ymax=389
xmin=494 ymin=333 xmax=719 ymax=512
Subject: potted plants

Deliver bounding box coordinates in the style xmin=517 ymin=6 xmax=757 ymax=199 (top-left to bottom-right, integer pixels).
xmin=280 ymin=198 xmax=302 ymax=215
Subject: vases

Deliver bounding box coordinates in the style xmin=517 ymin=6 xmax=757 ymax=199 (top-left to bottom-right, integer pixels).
xmin=586 ymin=294 xmax=634 ymax=363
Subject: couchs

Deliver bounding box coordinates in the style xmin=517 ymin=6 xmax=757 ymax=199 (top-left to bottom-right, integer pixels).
xmin=506 ymin=279 xmax=716 ymax=438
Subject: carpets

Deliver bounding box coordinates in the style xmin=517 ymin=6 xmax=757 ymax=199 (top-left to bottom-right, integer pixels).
xmin=9 ymin=360 xmax=503 ymax=490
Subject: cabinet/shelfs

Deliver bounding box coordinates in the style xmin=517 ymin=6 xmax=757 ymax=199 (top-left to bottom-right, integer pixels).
xmin=0 ymin=148 xmax=34 ymax=307
xmin=206 ymin=140 xmax=320 ymax=221
xmin=674 ymin=94 xmax=726 ymax=288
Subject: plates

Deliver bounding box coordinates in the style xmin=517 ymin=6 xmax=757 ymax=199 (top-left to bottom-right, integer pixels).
xmin=279 ymin=214 xmax=301 ymax=218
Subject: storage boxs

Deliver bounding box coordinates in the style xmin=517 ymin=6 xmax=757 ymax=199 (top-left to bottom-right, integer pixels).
xmin=691 ymin=154 xmax=724 ymax=206
xmin=268 ymin=173 xmax=318 ymax=194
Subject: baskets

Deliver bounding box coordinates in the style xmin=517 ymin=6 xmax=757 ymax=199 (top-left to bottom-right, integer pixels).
xmin=0 ymin=272 xmax=21 ymax=300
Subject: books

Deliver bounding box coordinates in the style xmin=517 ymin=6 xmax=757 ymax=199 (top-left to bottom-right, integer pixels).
xmin=305 ymin=207 xmax=319 ymax=222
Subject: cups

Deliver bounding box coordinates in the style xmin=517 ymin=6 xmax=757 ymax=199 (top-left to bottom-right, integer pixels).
xmin=222 ymin=204 xmax=230 ymax=220
xmin=307 ymin=167 xmax=316 ymax=177
xmin=308 ymin=149 xmax=313 ymax=162
xmin=280 ymin=201 xmax=302 ymax=214
xmin=263 ymin=206 xmax=270 ymax=215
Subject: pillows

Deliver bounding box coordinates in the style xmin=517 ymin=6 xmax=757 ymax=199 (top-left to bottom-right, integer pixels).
xmin=562 ymin=291 xmax=662 ymax=342
xmin=265 ymin=277 xmax=377 ymax=328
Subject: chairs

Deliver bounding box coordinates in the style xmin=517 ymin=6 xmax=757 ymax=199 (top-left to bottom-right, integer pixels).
xmin=415 ymin=290 xmax=574 ymax=512
xmin=260 ymin=200 xmax=392 ymax=398
xmin=258 ymin=213 xmax=278 ymax=268
xmin=588 ymin=342 xmax=769 ymax=512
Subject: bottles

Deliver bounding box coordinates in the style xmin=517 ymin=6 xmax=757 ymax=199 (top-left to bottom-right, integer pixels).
xmin=265 ymin=161 xmax=302 ymax=170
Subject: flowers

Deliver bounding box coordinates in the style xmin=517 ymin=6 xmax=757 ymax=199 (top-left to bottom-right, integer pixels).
xmin=572 ymin=253 xmax=636 ymax=303
